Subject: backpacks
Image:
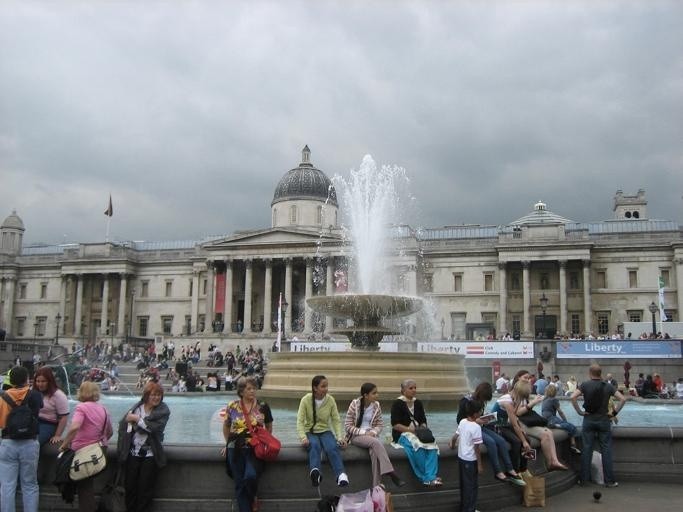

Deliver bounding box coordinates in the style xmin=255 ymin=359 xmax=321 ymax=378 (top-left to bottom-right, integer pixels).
xmin=0 ymin=390 xmax=39 ymax=441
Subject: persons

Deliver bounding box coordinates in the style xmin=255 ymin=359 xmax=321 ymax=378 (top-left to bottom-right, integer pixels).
xmin=2 ymin=338 xmax=270 ymax=392
xmin=0 ymin=363 xmax=42 ymax=511
xmin=210 ymin=316 xmax=333 ymax=354
xmin=344 ymin=382 xmax=405 ymax=494
xmin=219 ymin=377 xmax=273 ymax=512
xmin=449 ymin=363 xmax=683 ymax=511
xmin=57 ymin=381 xmax=114 ymax=511
xmin=446 ymin=328 xmax=678 ymax=344
xmin=15 ymin=367 xmax=71 ymax=496
xmin=296 ymin=375 xmax=351 ymax=488
xmin=117 ymin=385 xmax=170 ymax=511
xmin=389 ymin=380 xmax=443 ymax=488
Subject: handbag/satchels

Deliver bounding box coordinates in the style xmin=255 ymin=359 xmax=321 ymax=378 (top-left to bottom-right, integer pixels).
xmin=415 ymin=428 xmax=434 ymax=444
xmin=69 ymin=443 xmax=107 ymax=480
xmin=520 ymin=471 xmax=545 ymax=507
xmin=518 ymin=409 xmax=545 ymax=427
xmin=336 ymin=489 xmax=373 ymax=512
xmin=371 ymin=485 xmax=386 ymax=512
xmin=248 ymin=427 xmax=281 ymax=459
xmin=101 ymin=483 xmax=126 ymax=512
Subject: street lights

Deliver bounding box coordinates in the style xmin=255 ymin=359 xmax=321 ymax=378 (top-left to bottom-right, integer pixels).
xmin=537 ymin=293 xmax=551 ymax=340
xmin=129 ymin=288 xmax=135 ymax=336
xmin=647 ymin=301 xmax=658 ymax=336
xmin=51 ymin=311 xmax=61 ymax=344
xmin=439 ymin=317 xmax=444 ymax=340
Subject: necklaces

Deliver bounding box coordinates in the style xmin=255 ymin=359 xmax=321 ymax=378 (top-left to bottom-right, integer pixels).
xmin=247 ymin=399 xmax=254 ymax=415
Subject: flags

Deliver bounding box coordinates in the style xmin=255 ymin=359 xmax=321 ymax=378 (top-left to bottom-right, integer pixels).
xmin=658 ymin=275 xmax=667 ymax=321
xmin=103 ymin=191 xmax=113 ymax=218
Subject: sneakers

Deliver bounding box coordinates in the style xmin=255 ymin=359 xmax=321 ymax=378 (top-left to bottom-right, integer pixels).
xmin=337 ymin=473 xmax=348 ymax=488
xmin=507 ymin=470 xmax=518 ymax=477
xmin=422 ymin=480 xmax=443 ymax=489
xmin=308 ymin=468 xmax=321 ymax=487
xmin=250 ymin=496 xmax=257 ymax=512
xmin=495 ymin=472 xmax=506 ymax=480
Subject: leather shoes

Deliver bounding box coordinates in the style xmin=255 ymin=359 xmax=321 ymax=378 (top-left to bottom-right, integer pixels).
xmin=392 ymin=477 xmax=405 ymax=488
